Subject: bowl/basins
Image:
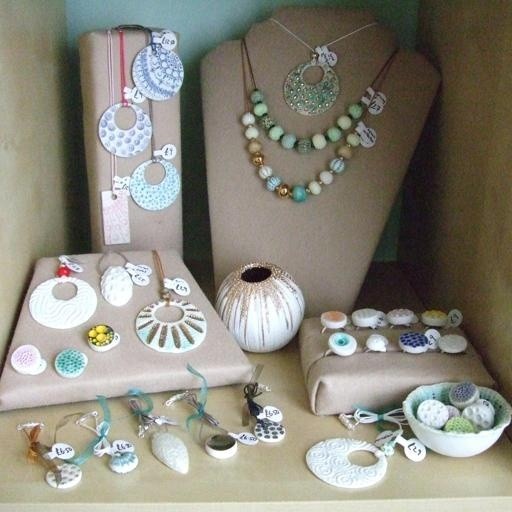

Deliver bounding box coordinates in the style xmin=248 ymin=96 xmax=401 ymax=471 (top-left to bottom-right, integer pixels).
xmin=403 ymin=380 xmax=511 ymax=457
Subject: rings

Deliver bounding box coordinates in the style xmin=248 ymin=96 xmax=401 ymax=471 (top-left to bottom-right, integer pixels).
xmin=319 ymin=305 xmax=470 ymax=357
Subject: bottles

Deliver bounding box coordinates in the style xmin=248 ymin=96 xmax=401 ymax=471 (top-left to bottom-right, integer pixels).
xmin=214 ymin=259 xmax=304 ymax=355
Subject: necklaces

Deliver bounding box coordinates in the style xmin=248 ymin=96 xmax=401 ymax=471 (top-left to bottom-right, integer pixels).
xmin=242 ymin=34 xmax=400 ymax=155
xmin=135 ymin=248 xmax=208 ymax=354
xmin=128 ymin=32 xmax=183 ymax=214
xmin=96 ymin=249 xmax=138 ymax=306
xmin=116 ymin=24 xmax=185 ymax=102
xmin=99 ymin=27 xmax=133 ymax=248
xmin=239 ymin=41 xmax=396 ymax=202
xmin=98 ymin=29 xmax=154 ymax=162
xmin=268 ymin=14 xmax=381 ymax=118
